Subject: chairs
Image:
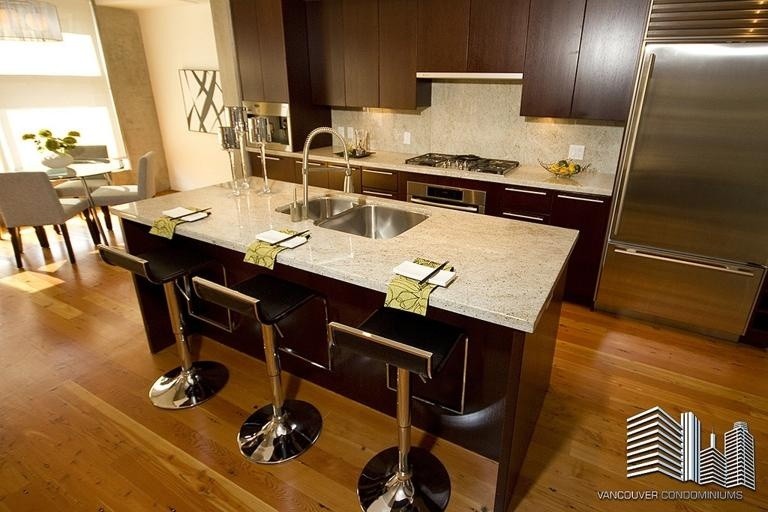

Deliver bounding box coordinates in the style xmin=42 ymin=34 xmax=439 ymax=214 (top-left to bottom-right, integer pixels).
xmin=0 ymin=145 xmax=156 ymax=268
xmin=192 ymin=273 xmax=332 ymax=464
xmin=328 ymin=307 xmax=470 ymax=512
xmin=97 ymin=243 xmax=240 ymax=410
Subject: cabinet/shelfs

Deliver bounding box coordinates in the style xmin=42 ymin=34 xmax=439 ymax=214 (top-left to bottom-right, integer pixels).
xmin=229 ymin=0 xmax=289 ymax=103
xmin=519 ymin=0 xmax=649 ymax=122
xmin=548 ymin=190 xmax=612 ymax=307
xmin=305 ymin=0 xmax=418 ymax=110
xmin=416 ymin=0 xmax=531 ymax=73
xmin=249 ymin=152 xmax=296 ymax=183
xmin=361 ymin=167 xmax=398 ymax=200
xmin=294 ymin=158 xmax=361 ymax=194
xmin=497 ymin=184 xmax=557 ymax=225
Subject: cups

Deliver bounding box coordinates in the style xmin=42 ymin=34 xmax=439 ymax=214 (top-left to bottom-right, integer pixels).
xmin=354 ymin=128 xmax=369 ymax=156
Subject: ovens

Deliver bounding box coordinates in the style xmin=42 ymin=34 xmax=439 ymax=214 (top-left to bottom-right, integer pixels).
xmin=406 ymin=180 xmax=486 ymax=215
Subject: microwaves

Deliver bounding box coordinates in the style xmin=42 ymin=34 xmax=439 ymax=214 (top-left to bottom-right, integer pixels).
xmin=240 ymin=101 xmax=294 ymax=153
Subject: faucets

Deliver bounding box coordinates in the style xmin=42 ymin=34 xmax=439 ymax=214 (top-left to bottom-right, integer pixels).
xmin=302 ymin=126 xmax=352 ymax=219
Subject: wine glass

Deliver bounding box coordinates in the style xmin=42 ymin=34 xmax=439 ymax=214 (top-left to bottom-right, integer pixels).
xmin=214 ymin=105 xmax=284 ymax=202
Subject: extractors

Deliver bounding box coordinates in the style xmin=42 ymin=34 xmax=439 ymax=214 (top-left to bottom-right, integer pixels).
xmin=414 ymin=71 xmax=524 ymax=85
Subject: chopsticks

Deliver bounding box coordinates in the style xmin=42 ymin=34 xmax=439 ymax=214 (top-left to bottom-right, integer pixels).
xmin=419 ymin=260 xmax=449 ymax=285
xmin=269 ymin=229 xmax=310 ymax=245
xmin=168 ymin=207 xmax=211 ymax=221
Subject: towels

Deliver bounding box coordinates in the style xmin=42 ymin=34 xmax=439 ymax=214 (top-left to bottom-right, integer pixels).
xmin=242 ymin=227 xmax=310 ymax=271
xmin=148 ymin=206 xmax=209 ymax=240
xmin=384 ymin=258 xmax=452 ymax=317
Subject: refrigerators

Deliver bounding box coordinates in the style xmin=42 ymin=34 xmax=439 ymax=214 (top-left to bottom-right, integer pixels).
xmin=595 ymin=39 xmax=767 ymax=344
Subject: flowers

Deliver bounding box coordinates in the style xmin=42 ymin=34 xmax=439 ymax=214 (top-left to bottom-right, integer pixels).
xmin=22 ymin=130 xmax=81 ymax=158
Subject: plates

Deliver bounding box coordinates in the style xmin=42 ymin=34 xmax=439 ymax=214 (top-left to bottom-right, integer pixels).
xmin=255 ymin=230 xmax=309 ymax=249
xmin=393 ymin=261 xmax=456 ymax=288
xmin=163 ymin=207 xmax=208 ymax=223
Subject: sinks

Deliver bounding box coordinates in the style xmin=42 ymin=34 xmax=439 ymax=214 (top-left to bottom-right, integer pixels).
xmin=276 ymin=193 xmax=366 ymax=219
xmin=318 ymin=205 xmax=432 ymax=241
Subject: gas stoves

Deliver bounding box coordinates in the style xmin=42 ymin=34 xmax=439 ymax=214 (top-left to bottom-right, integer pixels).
xmin=404 ymin=150 xmax=519 ymax=175
